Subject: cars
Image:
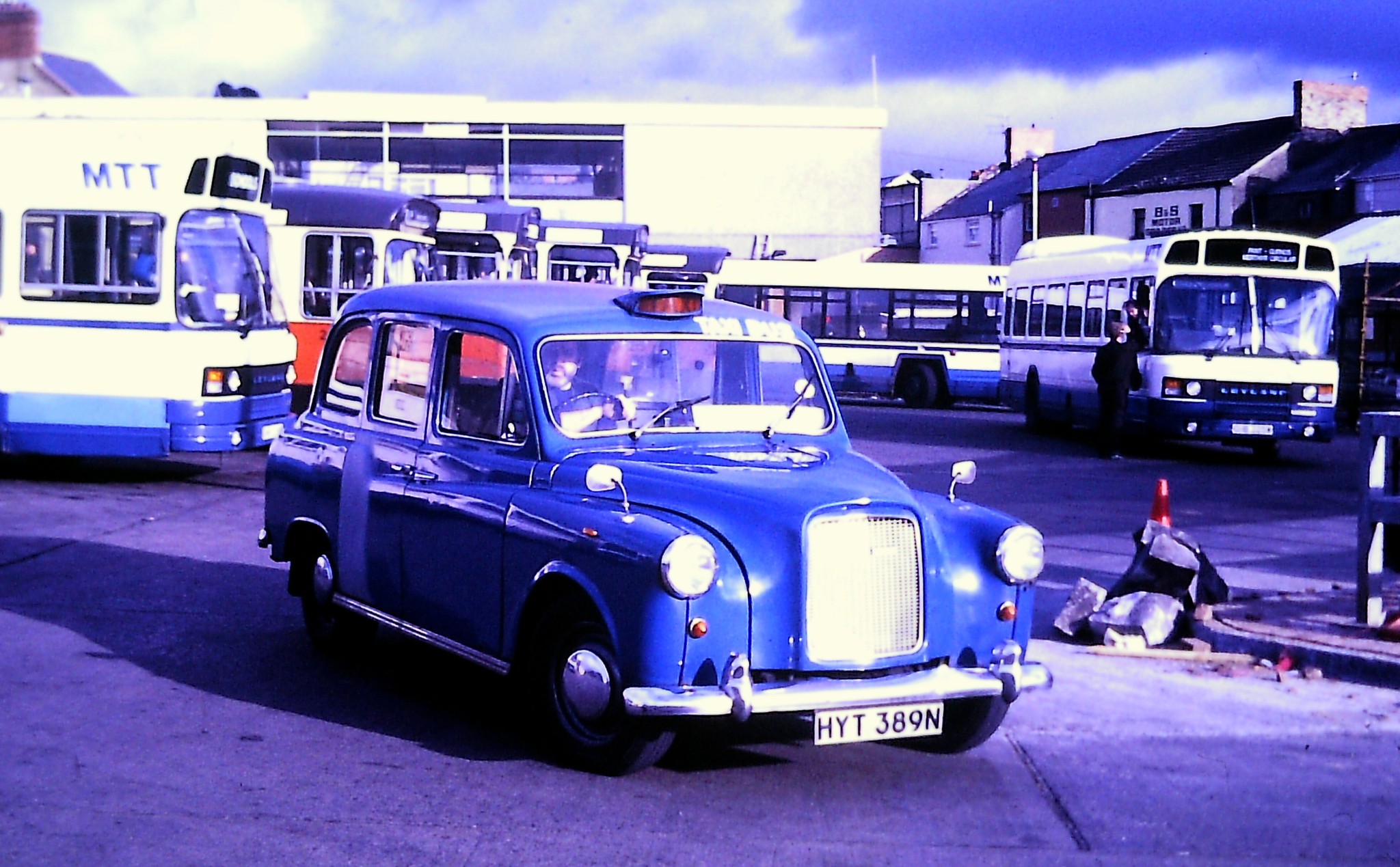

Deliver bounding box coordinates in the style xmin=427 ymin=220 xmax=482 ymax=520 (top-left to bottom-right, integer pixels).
xmin=258 ymin=270 xmax=1056 ymax=774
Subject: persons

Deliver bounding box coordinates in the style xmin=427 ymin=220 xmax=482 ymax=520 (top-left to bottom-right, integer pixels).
xmin=1091 ymin=322 xmax=1140 ymax=460
xmin=583 ymin=269 xmax=599 ymax=284
xmin=494 ymin=339 xmax=637 ymax=443
xmin=1124 ymin=300 xmax=1150 ymax=321
xmin=130 ymin=248 xmax=158 ymax=287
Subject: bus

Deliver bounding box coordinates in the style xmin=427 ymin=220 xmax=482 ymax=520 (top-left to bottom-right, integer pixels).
xmin=0 ymin=154 xmax=297 ymax=475
xmin=643 ymin=254 xmax=1011 ymax=409
xmin=420 ymin=195 xmax=731 ymax=300
xmin=995 ymin=229 xmax=1343 ymax=460
xmin=244 ymin=180 xmax=443 ymax=407
